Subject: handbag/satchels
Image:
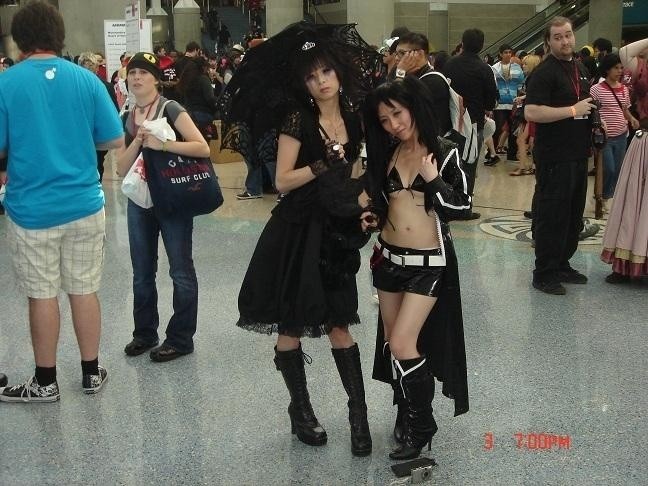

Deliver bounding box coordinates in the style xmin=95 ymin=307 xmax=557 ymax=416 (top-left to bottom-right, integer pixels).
xmin=120 ymin=141 xmax=225 ymax=226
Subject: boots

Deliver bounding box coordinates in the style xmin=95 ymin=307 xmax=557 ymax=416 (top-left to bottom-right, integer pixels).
xmin=273 ymin=339 xmax=330 ymax=447
xmin=329 ymin=342 xmax=374 ymax=458
xmin=379 ymin=338 xmax=438 ymax=460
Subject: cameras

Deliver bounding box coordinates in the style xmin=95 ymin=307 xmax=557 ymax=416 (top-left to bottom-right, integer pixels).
xmin=580 ymin=99 xmax=602 ymax=128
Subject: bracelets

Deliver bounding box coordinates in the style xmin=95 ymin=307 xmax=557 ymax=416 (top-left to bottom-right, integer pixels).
xmin=162 ymin=141 xmax=167 ymax=152
xmin=570 ymin=106 xmax=575 ymax=115
xmin=136 ymin=136 xmax=143 ymax=140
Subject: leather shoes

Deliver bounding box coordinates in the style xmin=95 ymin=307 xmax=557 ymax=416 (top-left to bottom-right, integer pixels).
xmin=149 ymin=339 xmax=195 ymax=363
xmin=123 ymin=332 xmax=160 ymax=356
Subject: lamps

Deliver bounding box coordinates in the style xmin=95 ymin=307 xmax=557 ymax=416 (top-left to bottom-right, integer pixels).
xmin=145 ymin=0 xmax=169 ymax=16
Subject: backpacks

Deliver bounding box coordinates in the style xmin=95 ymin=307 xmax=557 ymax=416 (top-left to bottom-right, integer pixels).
xmin=445 ymin=86 xmax=482 ymax=165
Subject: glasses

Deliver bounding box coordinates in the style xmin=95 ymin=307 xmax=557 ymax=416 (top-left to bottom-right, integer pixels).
xmin=393 ymin=49 xmax=420 ymax=57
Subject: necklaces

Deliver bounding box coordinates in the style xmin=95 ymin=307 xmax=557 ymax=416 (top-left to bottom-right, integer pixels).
xmin=135 ymin=95 xmax=161 ymax=113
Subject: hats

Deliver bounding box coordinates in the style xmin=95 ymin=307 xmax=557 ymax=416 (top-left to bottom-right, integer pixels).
xmin=497 ymin=44 xmax=513 ymax=61
xmin=93 ymin=51 xmax=162 ymax=83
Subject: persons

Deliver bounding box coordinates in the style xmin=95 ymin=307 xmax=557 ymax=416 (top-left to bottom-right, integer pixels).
xmin=577 ymin=38 xmax=640 ymax=217
xmin=1 ymin=38 xmax=266 ymax=200
xmin=601 ymin=37 xmax=648 ymax=292
xmin=376 ymin=25 xmax=543 ymax=220
xmin=234 ymin=35 xmax=385 ymax=460
xmin=0 ymin=0 xmax=123 ymax=403
xmin=523 ymin=14 xmax=604 ymax=296
xmin=356 ymin=73 xmax=476 ymax=463
xmin=115 ymin=51 xmax=203 ymax=365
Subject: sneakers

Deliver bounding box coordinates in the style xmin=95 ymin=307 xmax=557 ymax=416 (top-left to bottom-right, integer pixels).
xmin=532 ymin=259 xmax=590 ymax=295
xmin=81 ymin=365 xmax=110 ymax=395
xmin=605 ymin=270 xmax=631 ymax=284
xmin=578 ymin=190 xmax=614 ymax=241
xmin=1 ymin=376 xmax=62 ymax=403
xmin=482 ymin=144 xmax=537 ymax=177
